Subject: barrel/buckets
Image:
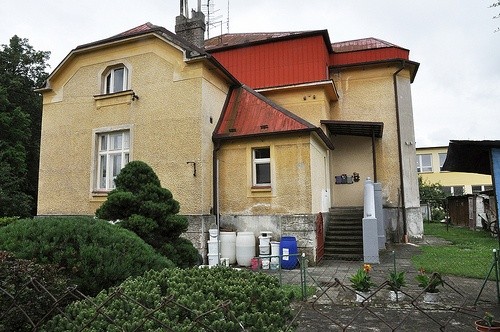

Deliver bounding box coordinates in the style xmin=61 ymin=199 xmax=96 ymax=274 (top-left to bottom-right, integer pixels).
xmin=261 ymin=257 xmax=280 ymax=270
xmin=219 ymin=232 xmax=236 ymax=264
xmin=258 ymin=236 xmax=272 ymax=245
xmin=236 ymin=232 xmax=255 ymax=266
xmin=271 ymin=242 xmax=279 ymax=256
xmin=259 ymin=244 xmax=270 ymax=254
xmin=280 ymin=236 xmax=297 ymax=270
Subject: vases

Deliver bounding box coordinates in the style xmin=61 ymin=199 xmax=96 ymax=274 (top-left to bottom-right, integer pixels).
xmin=316 ymin=283 xmax=341 ymax=299
xmin=355 ymin=290 xmax=372 ymax=303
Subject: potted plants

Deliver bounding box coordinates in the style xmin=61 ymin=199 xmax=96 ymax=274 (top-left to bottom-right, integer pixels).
xmin=474 ymin=312 xmax=500 ymax=332
xmin=415 ymin=274 xmax=441 ymax=301
xmin=387 ymin=271 xmax=407 ymax=301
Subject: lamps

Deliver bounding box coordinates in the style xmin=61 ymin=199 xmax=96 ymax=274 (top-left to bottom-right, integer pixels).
xmin=131 ymin=92 xmax=139 ymax=101
xmin=352 ymin=173 xmax=360 ymax=181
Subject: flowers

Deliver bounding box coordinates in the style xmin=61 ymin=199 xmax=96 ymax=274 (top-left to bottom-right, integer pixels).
xmin=347 ymin=264 xmax=379 ymax=292
